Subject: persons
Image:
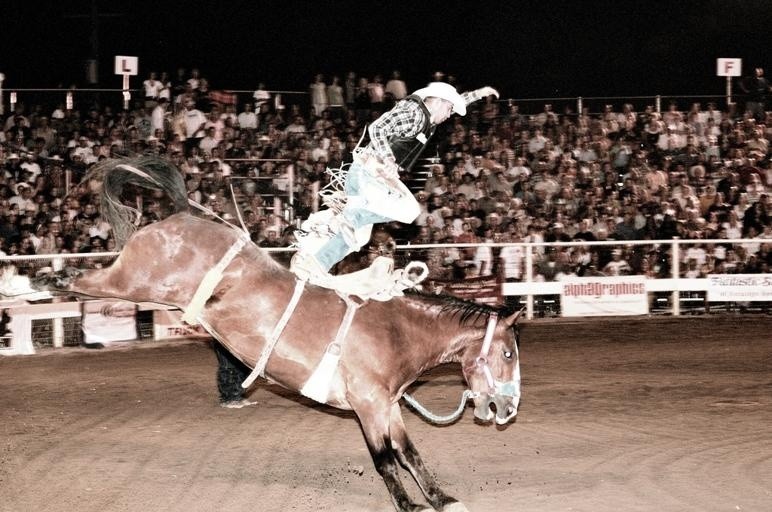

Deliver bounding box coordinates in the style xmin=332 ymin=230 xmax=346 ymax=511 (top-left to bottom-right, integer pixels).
xmin=1 ymin=68 xmax=771 ymax=308
xmin=215 ymin=334 xmax=259 ymax=410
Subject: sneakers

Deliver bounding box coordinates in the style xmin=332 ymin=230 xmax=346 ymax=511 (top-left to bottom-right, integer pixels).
xmin=220 ymin=400 xmax=245 ymax=409
xmin=241 ymin=397 xmax=259 ymax=407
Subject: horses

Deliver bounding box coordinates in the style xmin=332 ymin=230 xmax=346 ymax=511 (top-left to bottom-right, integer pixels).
xmin=0 ymin=153 xmax=527 ymax=512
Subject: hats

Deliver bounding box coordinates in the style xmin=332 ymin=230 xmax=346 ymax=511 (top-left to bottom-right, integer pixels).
xmin=412 ymin=81 xmax=468 ymax=119
